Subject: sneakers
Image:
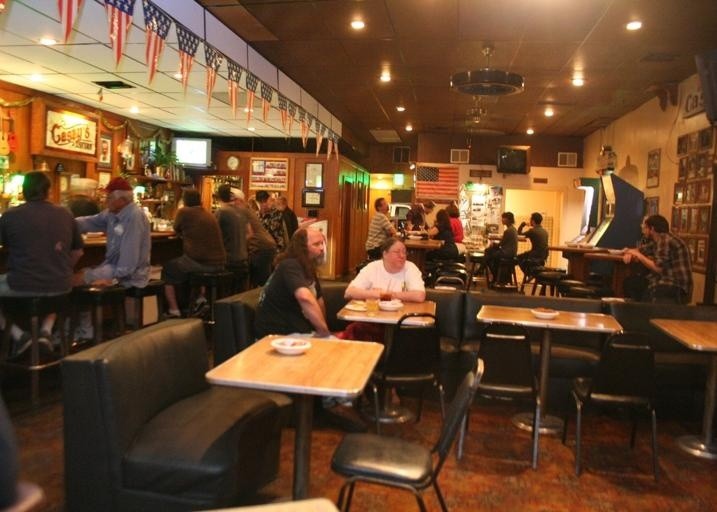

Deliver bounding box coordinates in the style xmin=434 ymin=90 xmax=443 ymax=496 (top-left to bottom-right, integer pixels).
xmin=358 ymin=425 xmax=385 ymax=437
xmin=7 ymin=332 xmax=33 ymax=361
xmin=158 ymin=308 xmax=182 ymax=319
xmin=53 ymin=323 xmax=95 ymax=346
xmin=37 ymin=330 xmax=54 ymax=353
xmin=191 ymin=300 xmax=210 ymax=318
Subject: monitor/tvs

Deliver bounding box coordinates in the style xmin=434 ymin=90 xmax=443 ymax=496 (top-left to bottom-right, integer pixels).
xmin=497 ymin=145 xmax=530 ymax=173
xmin=587 ymin=219 xmax=611 ymax=244
xmin=172 ymin=137 xmax=212 ymax=167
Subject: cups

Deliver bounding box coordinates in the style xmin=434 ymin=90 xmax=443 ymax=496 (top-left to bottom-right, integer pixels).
xmin=366 ymin=289 xmax=380 ymax=317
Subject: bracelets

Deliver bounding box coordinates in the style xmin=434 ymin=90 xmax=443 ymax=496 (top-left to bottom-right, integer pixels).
xmin=110 ymin=278 xmax=119 ymax=285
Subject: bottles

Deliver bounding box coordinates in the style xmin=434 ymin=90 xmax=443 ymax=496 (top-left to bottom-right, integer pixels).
xmin=212 ymin=175 xmax=241 ymax=209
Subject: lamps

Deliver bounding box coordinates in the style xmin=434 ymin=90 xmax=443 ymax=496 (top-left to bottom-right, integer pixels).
xmin=444 ymin=43 xmax=524 ymax=129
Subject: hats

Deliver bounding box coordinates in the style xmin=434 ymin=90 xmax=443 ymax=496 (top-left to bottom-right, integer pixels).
xmin=256 ymin=190 xmax=270 ymax=200
xmin=215 ymin=184 xmax=233 ymax=194
xmin=100 ymin=177 xmax=133 ymax=192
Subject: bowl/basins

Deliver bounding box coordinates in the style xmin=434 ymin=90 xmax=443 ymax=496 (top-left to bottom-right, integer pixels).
xmin=86 ymin=232 xmax=105 ymax=239
xmin=379 ymin=301 xmax=404 ymax=311
xmin=531 ymin=308 xmax=561 ymax=321
xmin=271 ymin=339 xmax=311 ymax=356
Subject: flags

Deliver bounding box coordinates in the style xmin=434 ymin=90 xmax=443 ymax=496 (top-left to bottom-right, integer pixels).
xmin=415 ymin=163 xmax=459 ymax=200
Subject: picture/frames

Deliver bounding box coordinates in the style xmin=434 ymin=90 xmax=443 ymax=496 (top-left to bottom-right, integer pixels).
xmin=302 ymin=187 xmax=325 ymax=209
xmin=640 ymin=122 xmax=717 ymax=232
xmin=95 ymin=134 xmax=113 ymax=173
xmin=304 ymin=162 xmax=323 ymax=189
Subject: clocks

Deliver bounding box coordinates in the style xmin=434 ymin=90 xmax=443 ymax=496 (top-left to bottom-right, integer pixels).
xmin=225 ymin=153 xmax=241 ymax=171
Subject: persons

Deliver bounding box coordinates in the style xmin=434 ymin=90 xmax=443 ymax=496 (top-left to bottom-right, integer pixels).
xmin=100 ymin=140 xmax=110 ymax=164
xmin=157 ymin=185 xmax=299 ymax=320
xmin=68 ymin=173 xmax=154 ymax=341
xmin=342 ymin=236 xmax=426 ymax=406
xmin=0 ymin=171 xmax=84 ymax=358
xmin=623 ymin=215 xmax=655 ymax=299
xmin=621 ymin=212 xmax=690 ymax=301
xmin=67 ymin=177 xmax=101 ymax=218
xmin=254 ymin=224 xmax=365 ymax=427
xmin=366 ymin=197 xmax=552 ymax=286
